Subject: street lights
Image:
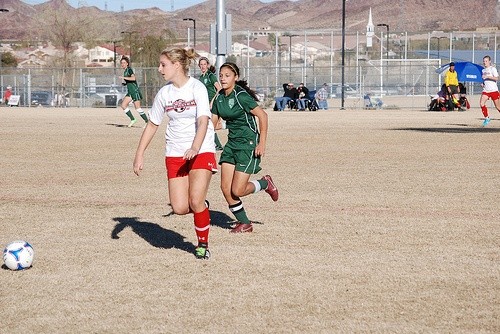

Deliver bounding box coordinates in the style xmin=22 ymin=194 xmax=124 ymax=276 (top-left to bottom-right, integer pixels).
xmin=430 ymin=36 xmax=447 ymax=58
xmin=106 ymin=40 xmax=124 ymax=95
xmin=394 ymin=44 xmax=405 ymax=83
xmin=472 ymin=33 xmax=476 ymax=95
xmin=401 ymin=32 xmax=407 ymax=95
xmin=282 ymin=34 xmax=301 ymax=83
xmin=182 ymin=17 xmax=197 ymax=79
xmin=377 ymin=24 xmax=389 ymax=96
xmin=120 ymin=31 xmax=141 ymax=63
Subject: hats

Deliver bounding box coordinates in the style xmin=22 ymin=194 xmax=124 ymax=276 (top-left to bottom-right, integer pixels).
xmin=450 ymin=62 xmax=455 ymax=66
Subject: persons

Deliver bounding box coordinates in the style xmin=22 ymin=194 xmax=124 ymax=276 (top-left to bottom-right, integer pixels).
xmin=317 ymin=83 xmax=329 ymax=110
xmin=118 ymin=57 xmax=148 ymax=128
xmin=479 ymin=56 xmax=500 ymax=126
xmin=275 ymin=83 xmax=313 ymax=111
xmin=132 ymin=47 xmax=217 ymax=260
xmin=198 ymin=57 xmax=224 ymax=151
xmin=374 ymin=97 xmax=383 ymax=110
xmin=211 ymin=62 xmax=278 ymax=233
xmin=427 ymin=62 xmax=470 ymax=111
xmin=364 ymin=93 xmax=373 ymax=110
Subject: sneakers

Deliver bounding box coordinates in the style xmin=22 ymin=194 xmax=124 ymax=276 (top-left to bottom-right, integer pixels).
xmin=229 ymin=222 xmax=252 ymax=234
xmin=261 ymin=175 xmax=278 ymax=202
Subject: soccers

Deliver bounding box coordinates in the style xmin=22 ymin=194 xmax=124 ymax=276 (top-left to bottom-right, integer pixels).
xmin=2 ymin=240 xmax=35 ymax=272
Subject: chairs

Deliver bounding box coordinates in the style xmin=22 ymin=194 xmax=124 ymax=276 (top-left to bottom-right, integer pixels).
xmin=281 ymin=91 xmax=319 ymax=111
xmin=365 ymin=99 xmax=370 ymax=107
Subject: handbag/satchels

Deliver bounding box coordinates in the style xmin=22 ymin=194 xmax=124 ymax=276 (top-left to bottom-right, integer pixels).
xmin=309 ymin=105 xmax=316 ymax=111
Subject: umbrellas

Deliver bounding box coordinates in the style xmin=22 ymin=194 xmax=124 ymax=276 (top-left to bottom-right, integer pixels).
xmin=435 ymin=61 xmax=486 ymax=88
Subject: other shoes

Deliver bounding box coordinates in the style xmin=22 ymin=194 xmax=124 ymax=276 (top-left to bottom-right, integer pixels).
xmin=128 ymin=117 xmax=137 ymax=127
xmin=482 ymin=117 xmax=491 ymax=126
xmin=305 ymin=108 xmax=309 ymax=112
xmin=195 ymin=246 xmax=210 ymax=262
xmin=222 ymin=119 xmax=226 ymax=129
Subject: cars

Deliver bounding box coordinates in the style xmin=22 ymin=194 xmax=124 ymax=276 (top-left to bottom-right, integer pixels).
xmin=252 ymin=82 xmax=446 ymax=102
xmin=0 ymin=85 xmax=134 ymax=108
